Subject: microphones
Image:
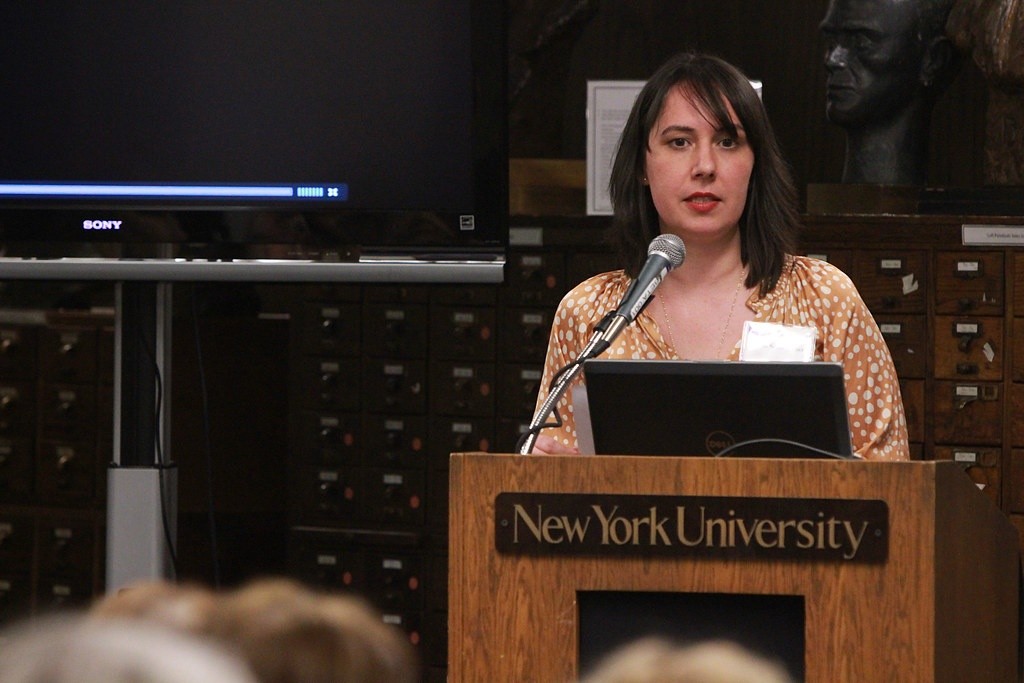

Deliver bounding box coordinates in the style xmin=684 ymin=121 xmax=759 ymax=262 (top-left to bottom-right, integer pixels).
xmin=590 ymin=234 xmax=686 ymax=357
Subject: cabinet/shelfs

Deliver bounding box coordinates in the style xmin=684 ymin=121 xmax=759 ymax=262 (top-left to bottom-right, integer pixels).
xmin=0 ymin=212 xmax=1024 ymax=683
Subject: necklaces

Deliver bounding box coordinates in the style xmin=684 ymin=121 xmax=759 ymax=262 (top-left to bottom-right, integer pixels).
xmin=660 ymin=270 xmax=745 ymax=359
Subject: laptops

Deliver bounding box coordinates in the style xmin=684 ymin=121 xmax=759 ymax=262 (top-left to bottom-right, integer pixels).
xmin=583 ymin=358 xmax=852 ymax=459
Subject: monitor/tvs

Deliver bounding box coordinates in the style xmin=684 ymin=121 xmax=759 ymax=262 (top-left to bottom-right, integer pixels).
xmin=0 ymin=0 xmax=510 ymax=247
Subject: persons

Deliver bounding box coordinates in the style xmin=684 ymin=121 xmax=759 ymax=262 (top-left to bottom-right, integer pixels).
xmin=524 ymin=53 xmax=909 ymax=462
xmin=1 ymin=580 xmax=792 ymax=682
xmin=816 ymin=0 xmax=953 ymax=187
